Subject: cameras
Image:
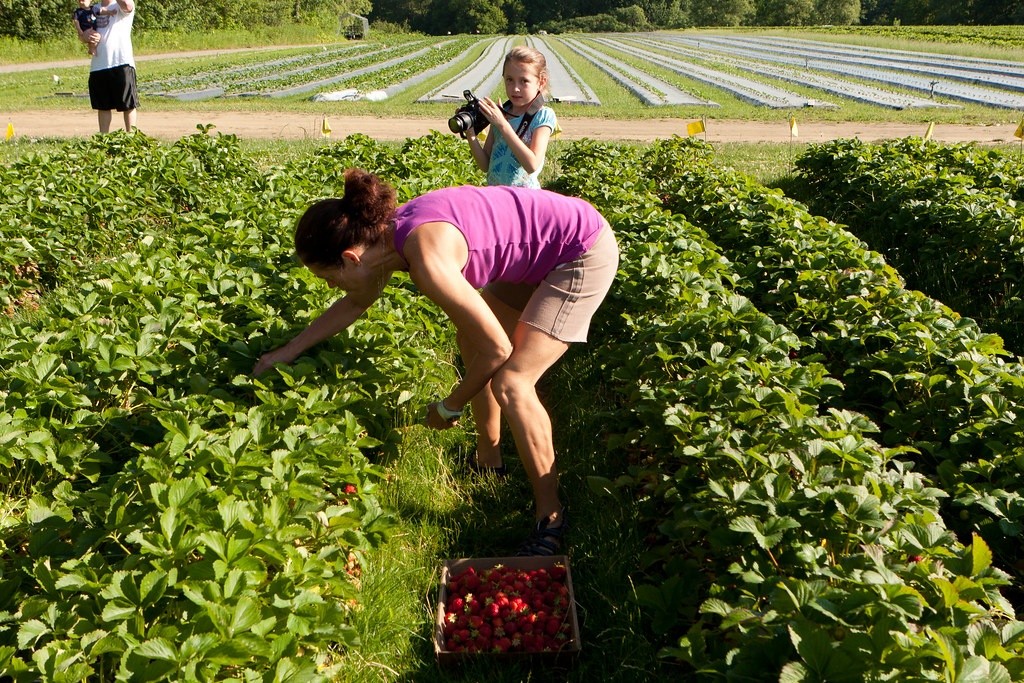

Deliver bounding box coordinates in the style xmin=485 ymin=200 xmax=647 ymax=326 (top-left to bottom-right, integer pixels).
xmin=448 ymin=89 xmax=491 ymax=133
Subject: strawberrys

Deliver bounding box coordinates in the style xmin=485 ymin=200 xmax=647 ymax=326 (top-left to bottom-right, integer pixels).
xmin=341 ymin=482 xmax=365 ymax=612
xmin=445 ymin=562 xmax=569 ymax=653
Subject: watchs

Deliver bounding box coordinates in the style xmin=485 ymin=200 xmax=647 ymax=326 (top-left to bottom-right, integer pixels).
xmin=437 ymin=398 xmax=462 ymax=422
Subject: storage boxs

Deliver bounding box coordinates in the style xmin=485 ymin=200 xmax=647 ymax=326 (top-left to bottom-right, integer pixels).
xmin=435 ymin=554 xmax=584 ymax=670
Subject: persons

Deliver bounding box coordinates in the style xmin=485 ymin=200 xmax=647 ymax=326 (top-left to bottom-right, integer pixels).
xmin=251 ymin=167 xmax=619 ymax=556
xmin=455 ymin=46 xmax=564 ymax=189
xmin=74 ymin=0 xmax=141 ymax=134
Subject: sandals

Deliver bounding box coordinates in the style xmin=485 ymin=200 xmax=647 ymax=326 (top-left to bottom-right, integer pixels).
xmin=455 ymin=456 xmax=504 ymax=475
xmin=512 ymin=519 xmax=565 ymax=556
